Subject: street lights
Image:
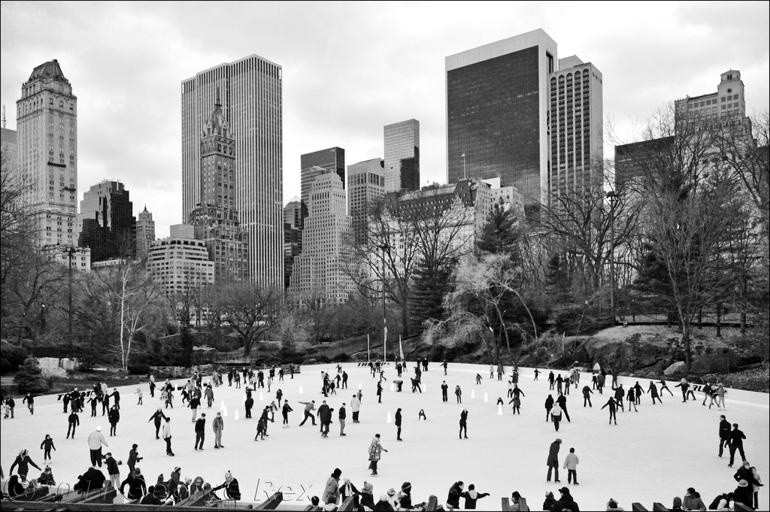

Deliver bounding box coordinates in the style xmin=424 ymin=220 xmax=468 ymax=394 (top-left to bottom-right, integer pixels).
xmin=377 ymin=242 xmax=395 ymax=363
xmin=63 ymin=245 xmax=79 ymax=357
xmin=604 ymin=189 xmax=621 ymax=326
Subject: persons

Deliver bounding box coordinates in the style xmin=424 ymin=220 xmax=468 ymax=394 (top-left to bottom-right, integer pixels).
xmin=508 ymin=360 xmax=525 ymax=415
xmin=533 ymin=369 xmax=541 ymax=380
xmin=490 ymin=360 xmax=505 ymax=381
xmin=227 ymin=365 xmax=295 ymax=441
xmin=547 ymin=359 xmax=581 ymax=397
xmin=591 ymin=367 xmax=619 ymax=394
xmin=0 ymin=434 xmax=240 ymax=508
xmin=476 ymin=373 xmax=483 ymax=384
xmin=717 ymin=414 xmax=746 ymax=467
xmin=299 ymin=358 xmax=468 ymax=442
xmin=601 ymin=377 xmax=728 ymax=426
xmin=22 ymin=391 xmax=34 ymax=415
xmin=305 ymin=461 xmax=764 ymax=511
xmin=497 ymin=397 xmax=504 ymax=407
xmin=134 ymin=369 xmax=224 ymax=456
xmin=58 ymin=380 xmax=120 ymax=439
xmin=1 ymin=395 xmax=15 ymax=418
xmin=545 ymin=392 xmax=571 ymax=432
xmin=368 ymin=435 xmax=388 ymax=476
xmin=547 ymin=438 xmax=579 ymax=485
xmin=582 ymin=385 xmax=594 ymax=408
